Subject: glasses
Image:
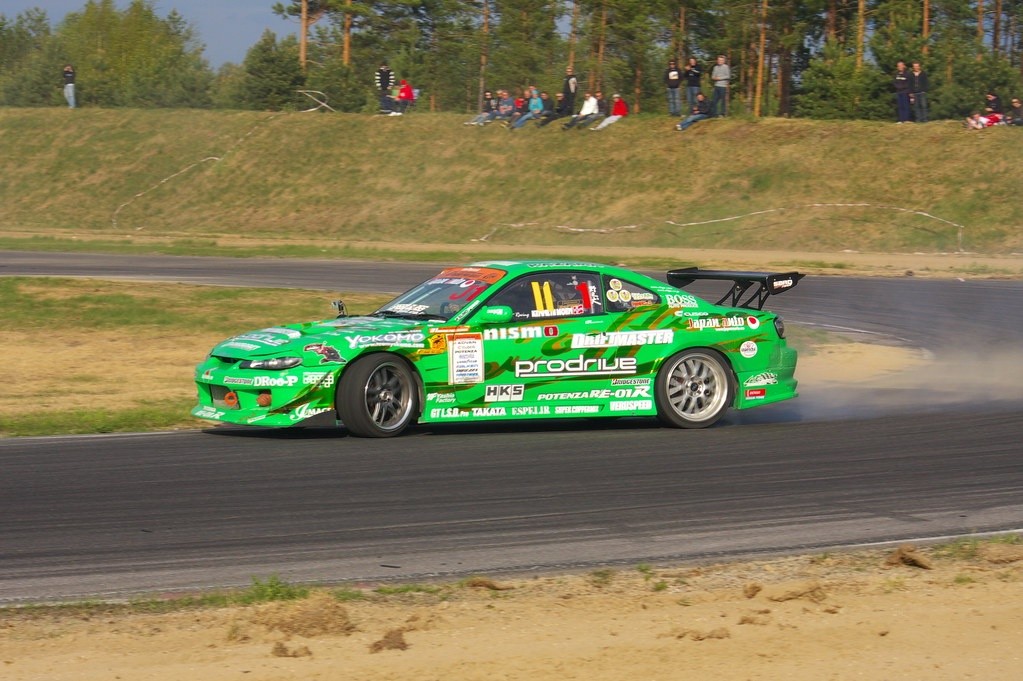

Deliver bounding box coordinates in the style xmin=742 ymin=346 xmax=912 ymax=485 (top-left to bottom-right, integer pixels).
xmin=669 ymin=62 xmax=675 ymax=65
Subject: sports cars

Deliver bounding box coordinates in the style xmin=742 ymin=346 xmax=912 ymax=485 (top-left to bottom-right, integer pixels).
xmin=192 ymin=256 xmax=806 ymax=445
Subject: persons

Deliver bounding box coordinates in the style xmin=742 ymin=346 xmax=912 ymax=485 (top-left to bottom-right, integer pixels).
xmin=559 ymin=90 xmax=629 ymax=133
xmin=462 ymin=66 xmax=578 ymax=134
xmin=374 ymin=60 xmax=414 ymax=117
xmin=448 ymin=287 xmax=532 ymax=320
xmin=964 ymin=90 xmax=1023 ymax=131
xmin=62 ymin=64 xmax=76 ymax=110
xmin=894 ymin=59 xmax=930 ymax=125
xmin=663 ymin=55 xmax=731 ymax=131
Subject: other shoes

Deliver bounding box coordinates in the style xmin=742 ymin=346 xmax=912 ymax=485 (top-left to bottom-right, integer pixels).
xmin=471 ymin=121 xmax=477 ymax=126
xmin=464 ymin=121 xmax=469 ymax=126
xmin=484 ymin=120 xmax=492 ymax=123
xmin=394 ymin=112 xmax=402 ymax=116
xmin=387 ymin=111 xmax=396 ymax=117
xmin=561 ymin=124 xmax=568 ymax=130
xmin=478 ymin=122 xmax=485 ymax=126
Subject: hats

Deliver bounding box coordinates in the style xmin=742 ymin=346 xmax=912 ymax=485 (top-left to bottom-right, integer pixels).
xmin=379 ymin=61 xmax=388 ymax=66
xmin=613 ymin=94 xmax=620 ymax=97
